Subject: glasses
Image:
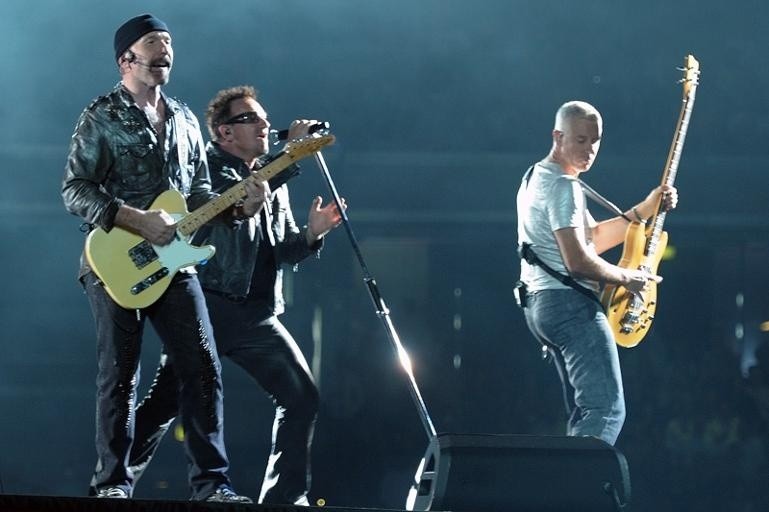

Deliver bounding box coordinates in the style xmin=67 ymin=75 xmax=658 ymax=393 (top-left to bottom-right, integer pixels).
xmin=225 ymin=110 xmax=258 ymax=125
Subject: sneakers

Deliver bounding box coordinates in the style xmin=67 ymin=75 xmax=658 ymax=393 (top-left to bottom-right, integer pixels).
xmin=98 ymin=487 xmax=130 ymax=500
xmin=205 ymin=484 xmax=252 ymax=503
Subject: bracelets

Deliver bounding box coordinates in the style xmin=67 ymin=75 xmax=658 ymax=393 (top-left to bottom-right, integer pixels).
xmin=632 ymin=206 xmax=646 ymax=223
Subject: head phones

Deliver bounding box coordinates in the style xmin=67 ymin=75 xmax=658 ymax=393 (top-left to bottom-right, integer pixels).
xmin=122 ymin=52 xmax=137 ymax=65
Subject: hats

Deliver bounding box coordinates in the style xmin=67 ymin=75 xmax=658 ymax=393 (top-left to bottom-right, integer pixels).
xmin=114 ymin=13 xmax=168 ymax=64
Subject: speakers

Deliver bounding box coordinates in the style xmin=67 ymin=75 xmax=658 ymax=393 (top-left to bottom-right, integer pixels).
xmin=405 ymin=431 xmax=632 ymax=511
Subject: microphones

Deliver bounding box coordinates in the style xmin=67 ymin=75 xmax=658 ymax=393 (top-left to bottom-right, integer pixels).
xmin=270 ymin=121 xmax=332 ymax=142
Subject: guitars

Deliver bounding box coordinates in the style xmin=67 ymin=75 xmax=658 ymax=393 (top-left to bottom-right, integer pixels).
xmin=84 ymin=136 xmax=333 ymax=310
xmin=600 ymin=56 xmax=701 ymax=350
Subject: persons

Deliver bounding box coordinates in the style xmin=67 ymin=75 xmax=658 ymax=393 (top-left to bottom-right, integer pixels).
xmin=60 ymin=15 xmax=254 ymax=503
xmin=515 ymin=100 xmax=678 ymax=445
xmin=90 ymin=85 xmax=346 ymax=507
